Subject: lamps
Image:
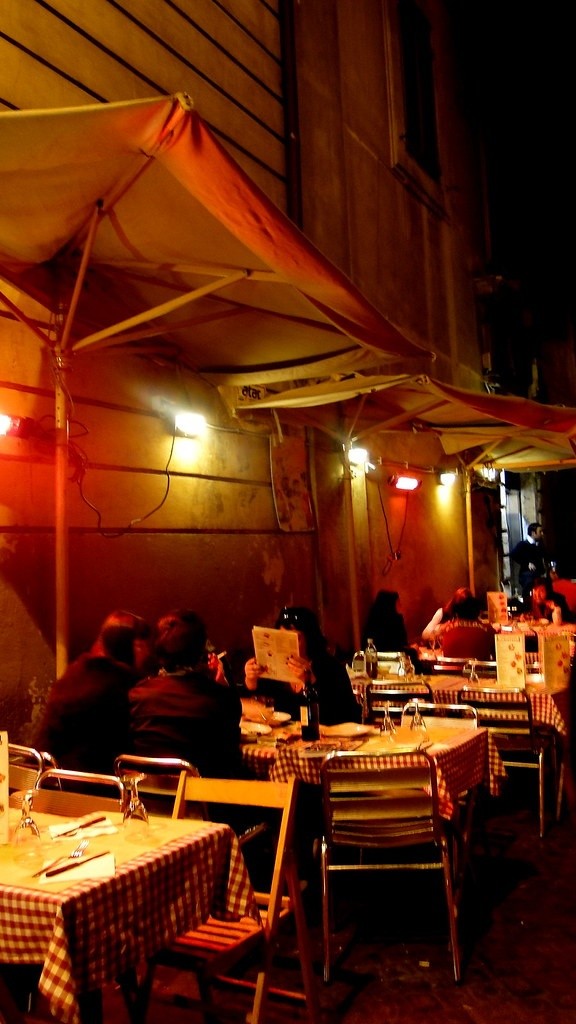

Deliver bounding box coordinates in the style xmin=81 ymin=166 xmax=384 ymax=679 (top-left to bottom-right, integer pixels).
xmin=389 ymin=474 xmax=423 ymax=491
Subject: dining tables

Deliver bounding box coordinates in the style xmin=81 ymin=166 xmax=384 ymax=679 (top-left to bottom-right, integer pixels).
xmin=501 ymin=620 xmax=576 ymax=638
xmin=241 ymin=720 xmax=509 ymax=894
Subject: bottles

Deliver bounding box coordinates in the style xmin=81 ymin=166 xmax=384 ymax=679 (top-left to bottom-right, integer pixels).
xmin=353 ymin=637 xmax=377 ymax=679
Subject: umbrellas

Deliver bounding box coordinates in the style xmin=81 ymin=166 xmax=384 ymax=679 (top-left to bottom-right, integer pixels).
xmin=0 ymin=92 xmax=475 ymax=700
xmin=234 ymin=374 xmax=576 ymax=648
xmin=434 ymin=431 xmax=576 ymax=594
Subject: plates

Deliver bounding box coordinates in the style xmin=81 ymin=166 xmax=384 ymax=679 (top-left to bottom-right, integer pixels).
xmin=239 ymin=711 xmax=292 ymax=741
xmin=298 ymin=666 xmax=320 ymax=741
xmin=320 ymin=721 xmax=371 ymax=737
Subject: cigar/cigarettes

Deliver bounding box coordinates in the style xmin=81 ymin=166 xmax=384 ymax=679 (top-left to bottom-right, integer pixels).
xmin=217 ymin=651 xmax=226 ymax=658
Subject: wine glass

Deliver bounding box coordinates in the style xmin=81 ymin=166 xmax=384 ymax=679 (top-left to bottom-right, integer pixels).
xmin=467 ymin=657 xmax=479 ymax=685
xmin=378 ymin=656 xmax=413 ymax=683
xmin=12 ymin=790 xmax=41 ymax=848
xmin=122 ymin=774 xmax=149 ymax=831
xmin=380 ymin=698 xmax=427 ymax=743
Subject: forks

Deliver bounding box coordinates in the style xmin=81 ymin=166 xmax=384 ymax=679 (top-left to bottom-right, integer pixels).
xmin=31 ymin=840 xmax=89 ymax=876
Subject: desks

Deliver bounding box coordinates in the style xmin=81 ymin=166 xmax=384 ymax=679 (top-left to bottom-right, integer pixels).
xmin=349 ymin=672 xmax=570 ymax=818
xmin=0 ymin=806 xmax=269 ymax=1024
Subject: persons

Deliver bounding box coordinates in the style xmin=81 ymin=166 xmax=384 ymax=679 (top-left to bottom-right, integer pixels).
xmin=511 ymin=523 xmax=576 ymax=622
xmin=243 ymin=605 xmax=362 ymax=725
xmin=422 ymin=589 xmax=496 ymax=675
xmin=24 ymin=608 xmax=267 ymax=834
xmin=366 ymin=590 xmax=432 ymax=676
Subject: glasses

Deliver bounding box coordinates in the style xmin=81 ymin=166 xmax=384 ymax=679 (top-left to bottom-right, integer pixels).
xmin=279 ymin=611 xmax=303 ymax=621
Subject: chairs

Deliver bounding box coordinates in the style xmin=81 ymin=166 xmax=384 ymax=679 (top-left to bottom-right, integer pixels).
xmin=8 ymin=651 xmax=576 ymax=1024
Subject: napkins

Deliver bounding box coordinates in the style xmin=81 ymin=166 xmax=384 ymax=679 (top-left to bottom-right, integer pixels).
xmin=49 ymin=817 xmax=116 ymax=842
xmin=39 ymin=851 xmax=114 ymax=882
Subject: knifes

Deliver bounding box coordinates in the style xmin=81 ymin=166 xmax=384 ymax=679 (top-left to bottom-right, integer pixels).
xmin=33 ymin=851 xmax=109 ymax=877
xmin=51 ymin=816 xmax=105 ymax=839
